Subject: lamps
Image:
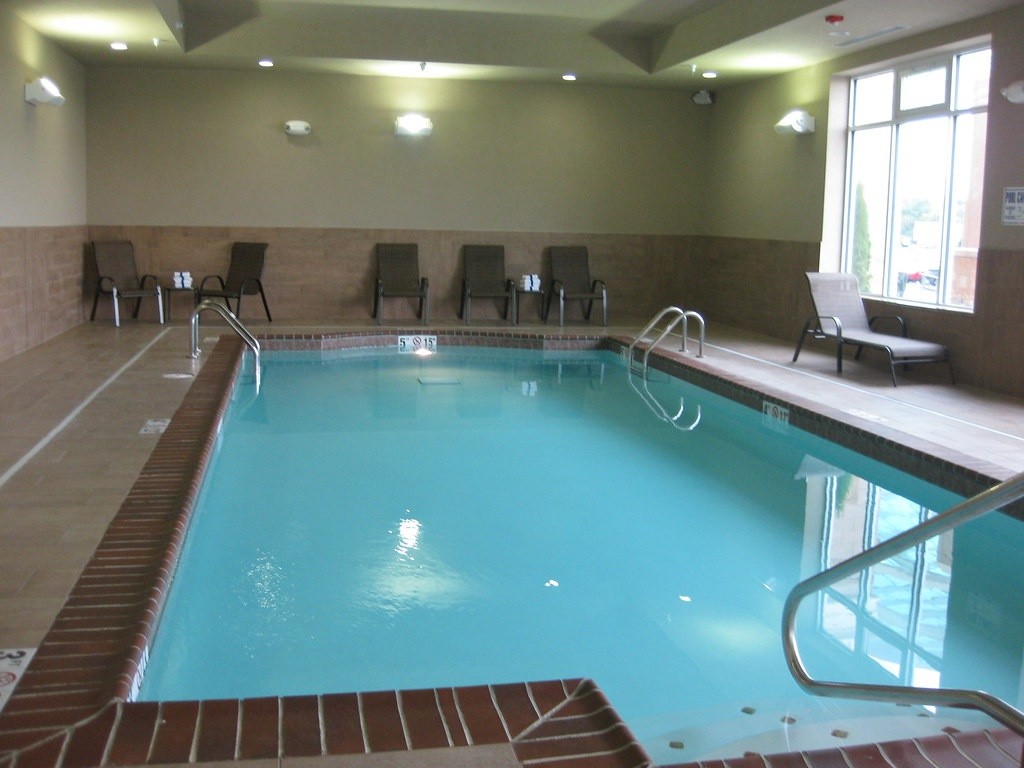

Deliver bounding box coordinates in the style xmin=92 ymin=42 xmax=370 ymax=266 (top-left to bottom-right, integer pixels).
xmin=25 ymin=77 xmax=66 ymax=110
xmin=1000 ymin=80 xmax=1024 ymax=104
xmin=773 ymin=109 xmax=817 ymax=136
xmin=393 ymin=112 xmax=433 ymax=137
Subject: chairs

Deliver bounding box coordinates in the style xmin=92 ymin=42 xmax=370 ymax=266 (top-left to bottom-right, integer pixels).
xmin=458 ymin=244 xmax=518 ymax=327
xmin=90 ymin=239 xmax=165 ymax=327
xmin=793 ymin=272 xmax=958 ymax=386
xmin=544 ymin=245 xmax=609 ymax=328
xmin=691 ymin=89 xmax=717 ymax=106
xmin=199 ymin=241 xmax=273 ymax=325
xmin=373 ymin=242 xmax=431 ymax=327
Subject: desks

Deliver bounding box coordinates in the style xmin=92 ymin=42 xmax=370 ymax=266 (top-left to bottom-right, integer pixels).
xmin=516 ymin=287 xmax=547 ymax=325
xmin=163 ymin=286 xmax=200 ymax=326
